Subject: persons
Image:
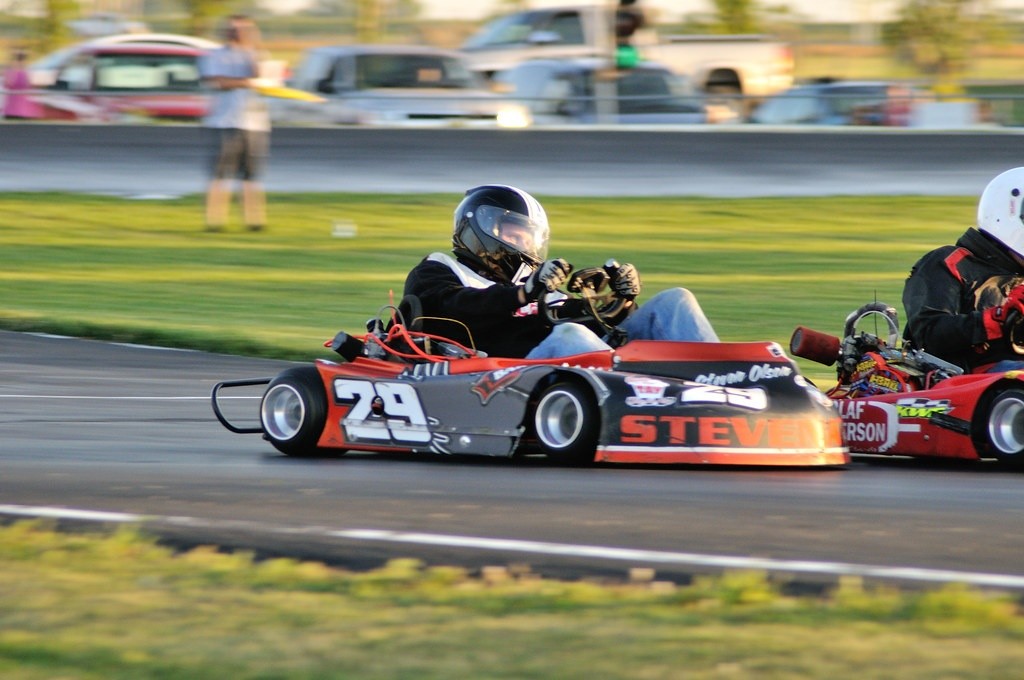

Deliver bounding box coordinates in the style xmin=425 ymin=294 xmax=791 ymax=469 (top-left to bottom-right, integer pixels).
xmin=405 ymin=184 xmax=719 ymax=361
xmin=903 ymin=167 xmax=1024 ymax=373
xmin=0 ymin=51 xmax=37 ymax=120
xmin=198 ymin=16 xmax=278 ymax=233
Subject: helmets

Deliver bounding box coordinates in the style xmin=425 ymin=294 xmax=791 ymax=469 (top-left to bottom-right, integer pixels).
xmin=977 ymin=167 xmax=1024 ymax=261
xmin=451 ymin=185 xmax=549 ymax=288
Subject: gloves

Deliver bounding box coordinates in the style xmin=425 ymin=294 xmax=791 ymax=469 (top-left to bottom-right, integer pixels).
xmin=603 ymin=260 xmax=640 ymax=311
xmin=991 ymin=284 xmax=1024 ymax=322
xmin=523 ymin=257 xmax=570 ymax=303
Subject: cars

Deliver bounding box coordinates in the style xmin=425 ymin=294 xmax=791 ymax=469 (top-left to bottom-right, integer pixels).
xmin=1 ymin=0 xmax=985 ymax=127
